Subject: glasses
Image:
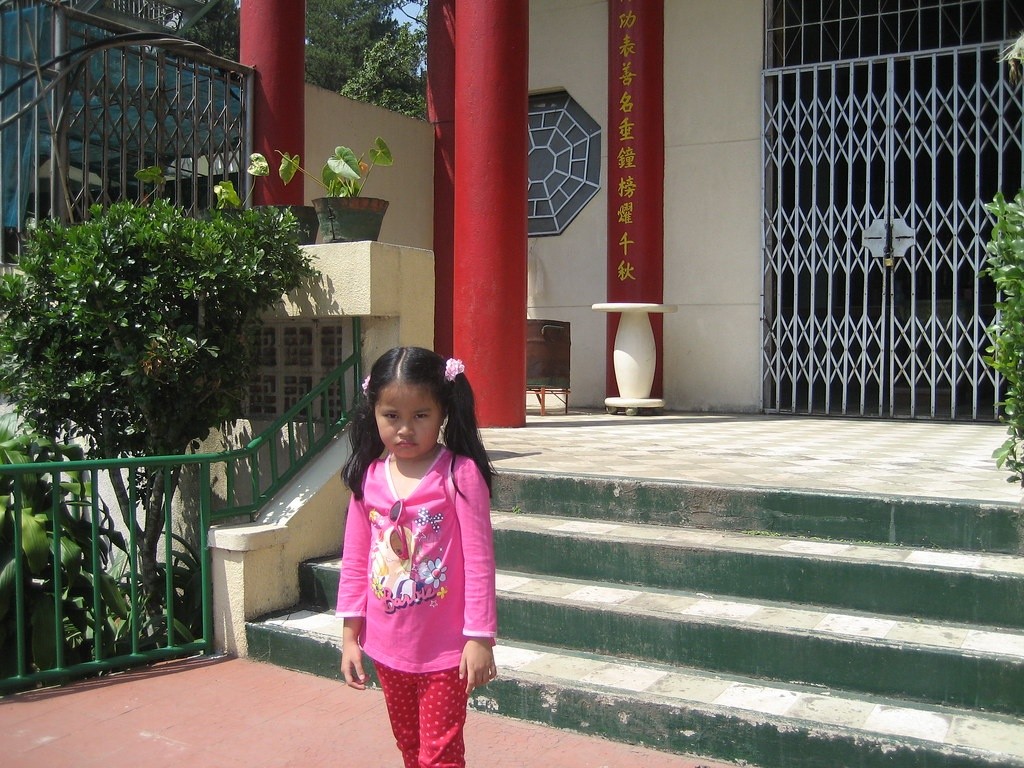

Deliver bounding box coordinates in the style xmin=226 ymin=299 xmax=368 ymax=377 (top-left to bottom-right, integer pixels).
xmin=388 ymin=499 xmax=409 ymax=560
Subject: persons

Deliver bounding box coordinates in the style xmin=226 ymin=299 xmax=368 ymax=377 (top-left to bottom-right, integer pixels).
xmin=336 ymin=345 xmax=499 ymax=768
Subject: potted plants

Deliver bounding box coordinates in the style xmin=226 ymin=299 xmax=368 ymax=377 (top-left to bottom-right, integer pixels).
xmin=263 ymin=151 xmax=320 ymax=245
xmin=274 ymin=135 xmax=394 ymax=242
xmin=210 ymin=154 xmax=269 ymax=244
